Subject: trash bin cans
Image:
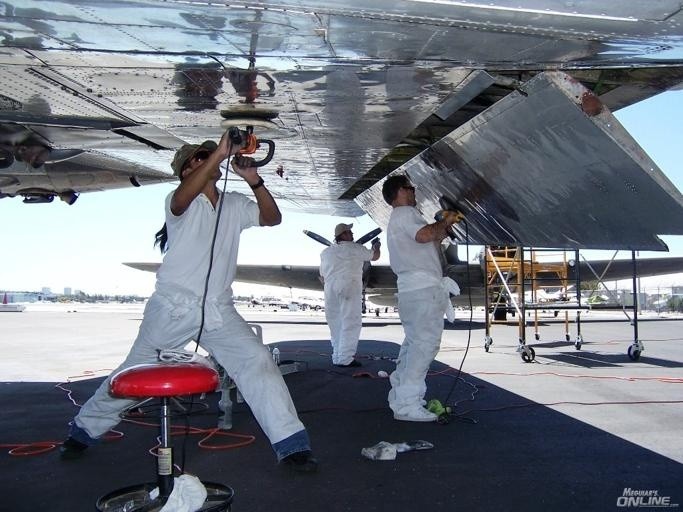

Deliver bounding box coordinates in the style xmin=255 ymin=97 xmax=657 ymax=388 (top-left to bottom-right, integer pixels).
xmin=493 ymin=297 xmax=506 ymax=320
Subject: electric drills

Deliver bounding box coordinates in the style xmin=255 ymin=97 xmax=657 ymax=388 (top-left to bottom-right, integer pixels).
xmin=228 ymin=125 xmax=275 ymax=167
xmin=434 ymin=207 xmax=462 ymax=239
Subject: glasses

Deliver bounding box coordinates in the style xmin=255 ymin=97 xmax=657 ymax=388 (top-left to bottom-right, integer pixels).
xmin=404 ymin=186 xmax=415 ymax=194
xmin=185 ymin=150 xmax=210 ymax=167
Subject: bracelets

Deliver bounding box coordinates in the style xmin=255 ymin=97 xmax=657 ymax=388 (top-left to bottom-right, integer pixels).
xmin=251 ymin=177 xmax=265 ymax=190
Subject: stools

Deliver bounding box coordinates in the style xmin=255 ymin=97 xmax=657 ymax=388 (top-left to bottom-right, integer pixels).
xmin=94 ymin=361 xmax=237 ymax=512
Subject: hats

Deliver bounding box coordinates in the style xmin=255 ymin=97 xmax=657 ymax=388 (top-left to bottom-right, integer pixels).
xmin=335 ymin=223 xmax=353 ymax=237
xmin=171 ymin=140 xmax=218 ymax=177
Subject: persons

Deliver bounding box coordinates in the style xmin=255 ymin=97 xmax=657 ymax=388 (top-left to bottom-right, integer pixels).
xmin=318 ymin=223 xmax=382 ymax=368
xmin=57 ymin=128 xmax=317 ymax=479
xmin=381 ymin=175 xmax=458 ymax=422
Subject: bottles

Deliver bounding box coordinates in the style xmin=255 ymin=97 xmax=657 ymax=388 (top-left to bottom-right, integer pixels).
xmin=272 ymin=346 xmax=280 ymax=367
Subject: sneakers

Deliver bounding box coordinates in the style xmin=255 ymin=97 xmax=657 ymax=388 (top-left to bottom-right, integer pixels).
xmin=280 ymin=450 xmax=322 ymax=473
xmin=60 ymin=437 xmax=89 ymax=460
xmin=394 ymin=406 xmax=438 ymax=422
xmin=333 ymin=359 xmax=363 ymax=368
